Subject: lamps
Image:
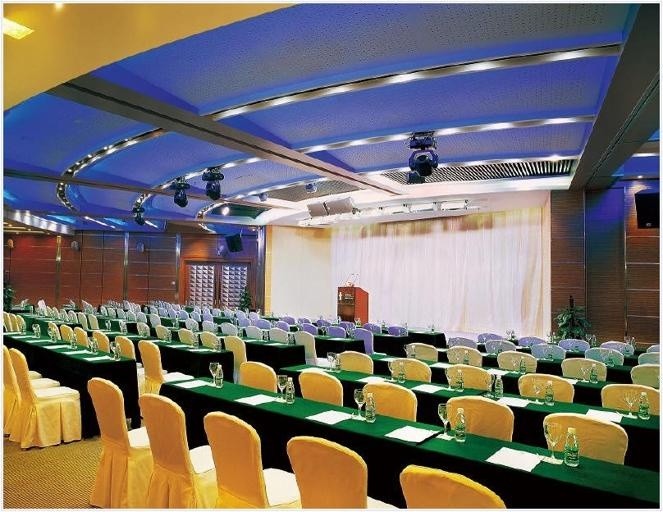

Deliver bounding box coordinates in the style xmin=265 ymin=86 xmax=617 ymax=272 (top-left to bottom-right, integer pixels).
xmin=404 ymin=129 xmax=443 ymax=178
xmin=201 ymin=164 xmax=225 ymax=202
xmin=131 ymin=205 xmax=147 ymax=227
xmin=168 ymin=177 xmax=192 ymax=209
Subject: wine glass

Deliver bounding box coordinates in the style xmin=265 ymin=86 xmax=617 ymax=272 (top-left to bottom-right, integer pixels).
xmin=208 ymin=361 xmax=219 ymax=388
xmin=326 ymin=352 xmax=334 ymax=374
xmin=437 ymin=403 xmax=454 ymax=437
xmin=353 ymin=388 xmax=366 ymax=419
xmin=276 ymin=375 xmax=286 ymax=401
xmin=541 ymin=422 xmax=561 ymax=462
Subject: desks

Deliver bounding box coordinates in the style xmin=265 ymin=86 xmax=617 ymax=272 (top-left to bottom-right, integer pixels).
xmin=273 ymin=362 xmax=657 ymax=471
xmin=5 ymin=323 xmax=143 ymax=441
xmin=155 ymin=371 xmax=658 ymax=509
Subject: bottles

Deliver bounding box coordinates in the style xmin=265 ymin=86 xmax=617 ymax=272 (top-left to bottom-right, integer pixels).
xmin=16 ymin=321 xmax=123 ymax=361
xmin=482 ymin=334 xmax=650 ymax=420
xmin=364 ymin=392 xmax=375 ymax=422
xmin=85 ymin=302 xmax=308 ymax=353
xmin=453 ymin=408 xmax=466 ymax=442
xmin=285 ymin=377 xmax=295 ymax=404
xmin=317 ymin=313 xmax=406 ymax=342
xmin=334 ymin=333 xmax=506 ymax=393
xmin=213 ymin=364 xmax=223 ymax=389
xmin=563 ymin=426 xmax=580 ymax=468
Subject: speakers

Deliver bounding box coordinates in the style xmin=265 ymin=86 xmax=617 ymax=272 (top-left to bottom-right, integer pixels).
xmin=633 ymin=188 xmax=660 ymax=230
xmin=224 ymin=233 xmax=243 ymax=252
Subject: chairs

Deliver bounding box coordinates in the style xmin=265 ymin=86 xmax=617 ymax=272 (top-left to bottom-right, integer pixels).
xmin=7 ymin=294 xmax=451 ymax=384
xmin=84 ymin=375 xmax=153 ymax=507
xmin=201 ymin=408 xmax=301 ymax=506
xmin=296 ymin=367 xmax=345 ymax=409
xmin=336 ymin=349 xmax=374 ymax=376
xmin=443 ymin=394 xmax=518 ymax=444
xmin=4 ymin=347 xmax=80 ymax=448
xmin=359 ymin=382 xmax=420 ymax=425
xmin=598 ymin=381 xmax=656 ymax=417
xmin=137 ymin=335 xmax=196 ymax=397
xmin=285 ymin=434 xmax=397 ymax=508
xmin=442 ymin=364 xmax=493 ymax=391
xmin=517 ymin=372 xmax=576 ymax=405
xmin=135 ymin=391 xmax=219 ymax=510
xmin=403 ymin=330 xmax=658 ymax=388
xmin=540 ymin=408 xmax=631 ymax=467
xmin=388 ymin=356 xmax=433 ymax=384
xmin=235 ymin=359 xmax=280 ymax=395
xmin=398 ymin=463 xmax=506 ymax=509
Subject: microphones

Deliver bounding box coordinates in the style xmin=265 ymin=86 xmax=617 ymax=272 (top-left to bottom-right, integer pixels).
xmin=345 ymin=272 xmax=354 ymax=288
xmin=353 ymin=273 xmax=360 ymax=286
